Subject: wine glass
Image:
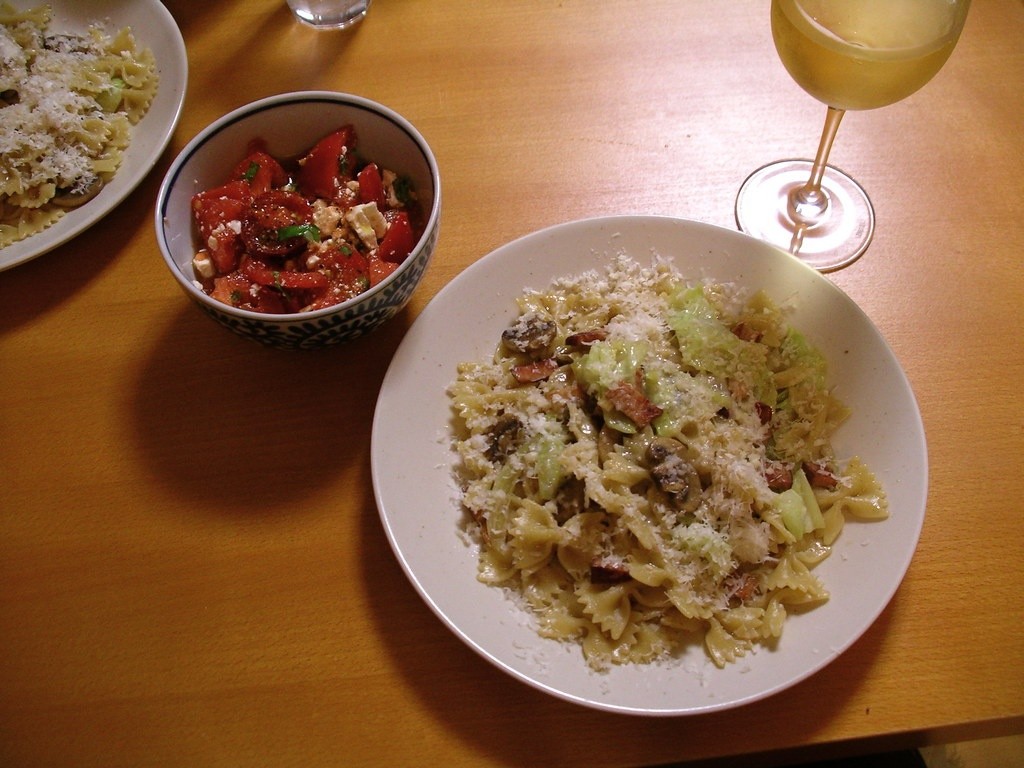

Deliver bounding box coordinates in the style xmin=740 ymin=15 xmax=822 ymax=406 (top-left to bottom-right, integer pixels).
xmin=735 ymin=1 xmax=972 ymax=272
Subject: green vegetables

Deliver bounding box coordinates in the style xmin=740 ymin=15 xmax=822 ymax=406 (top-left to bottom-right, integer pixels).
xmin=244 ymin=147 xmax=413 ymax=254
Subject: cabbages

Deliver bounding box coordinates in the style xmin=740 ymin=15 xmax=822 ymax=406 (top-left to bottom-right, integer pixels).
xmin=483 ymin=278 xmax=830 ymax=542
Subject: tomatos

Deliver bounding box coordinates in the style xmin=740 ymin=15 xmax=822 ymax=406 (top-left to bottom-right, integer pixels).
xmin=190 ymin=124 xmax=420 ymax=315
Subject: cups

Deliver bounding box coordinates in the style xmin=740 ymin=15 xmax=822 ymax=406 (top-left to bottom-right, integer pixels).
xmin=286 ymin=0 xmax=371 ymax=30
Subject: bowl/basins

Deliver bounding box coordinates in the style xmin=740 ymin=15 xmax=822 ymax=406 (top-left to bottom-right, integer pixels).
xmin=154 ymin=91 xmax=441 ymax=353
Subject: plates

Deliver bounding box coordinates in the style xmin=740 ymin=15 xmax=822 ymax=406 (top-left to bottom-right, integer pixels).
xmin=371 ymin=214 xmax=927 ymax=716
xmin=0 ymin=1 xmax=189 ymax=271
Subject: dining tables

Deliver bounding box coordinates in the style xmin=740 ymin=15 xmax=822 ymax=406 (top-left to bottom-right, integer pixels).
xmin=0 ymin=0 xmax=1024 ymax=768
